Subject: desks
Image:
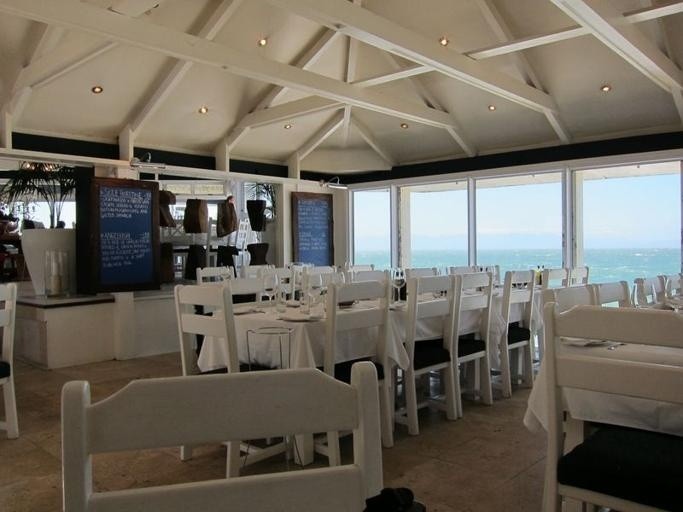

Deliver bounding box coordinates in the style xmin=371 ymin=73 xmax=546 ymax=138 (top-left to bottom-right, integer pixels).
xmin=159 ymin=230 xmax=265 ymax=247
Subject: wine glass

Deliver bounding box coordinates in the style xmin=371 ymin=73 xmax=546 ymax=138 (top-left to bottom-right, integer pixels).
xmin=630 ymin=284 xmax=644 ymax=308
xmin=438 ymin=266 xmax=450 ymax=299
xmin=391 ymin=268 xmax=407 ymax=307
xmin=306 ymin=272 xmax=322 ymax=312
xmin=330 ymin=272 xmax=344 ymax=311
xmin=263 ymin=271 xmax=279 ymax=316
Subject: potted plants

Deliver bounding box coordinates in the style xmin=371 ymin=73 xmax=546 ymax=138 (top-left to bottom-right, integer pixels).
xmin=0 ymin=159 xmax=81 ymax=297
xmin=242 ymin=180 xmax=278 ymax=266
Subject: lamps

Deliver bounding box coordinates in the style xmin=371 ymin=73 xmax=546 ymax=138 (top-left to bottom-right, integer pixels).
xmin=320 ymin=176 xmax=349 ymax=190
xmin=130 ymin=152 xmax=167 ymax=172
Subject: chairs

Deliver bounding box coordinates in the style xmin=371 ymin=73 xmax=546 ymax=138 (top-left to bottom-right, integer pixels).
xmin=632 ymin=276 xmax=663 ymax=306
xmin=404 ymin=267 xmax=439 ymax=278
xmin=531 ymin=268 xmax=570 ymax=369
xmin=570 ymin=266 xmax=588 ymax=286
xmin=392 ymin=276 xmax=457 ymax=436
xmin=452 ymin=271 xmax=494 ymax=419
xmin=255 ymin=269 xmax=295 ymax=301
xmin=302 ymin=265 xmax=337 ymax=296
xmin=0 ymin=282 xmax=19 ymax=439
xmin=345 ymin=265 xmax=370 ymax=271
xmin=241 ymin=265 xmax=276 ymax=278
xmin=313 ymin=270 xmax=394 ymax=468
xmin=476 ymin=265 xmax=499 ymax=285
xmin=60 ymin=361 xmax=384 ymax=512
xmin=231 ymin=219 xmax=250 ymax=269
xmin=344 ymin=269 xmax=391 ymax=285
xmin=496 ymin=270 xmax=536 ymax=399
xmin=206 ymin=217 xmax=217 ymax=268
xmin=663 ymin=273 xmax=683 ymax=303
xmin=592 ymin=280 xmax=629 ymax=309
xmin=196 ymin=266 xmax=236 ymax=285
xmin=542 ymin=284 xmax=598 ymax=314
xmin=446 ymin=266 xmax=477 ymax=276
xmin=540 ymin=301 xmax=683 ymax=512
xmin=173 ymin=284 xmax=294 ymax=479
